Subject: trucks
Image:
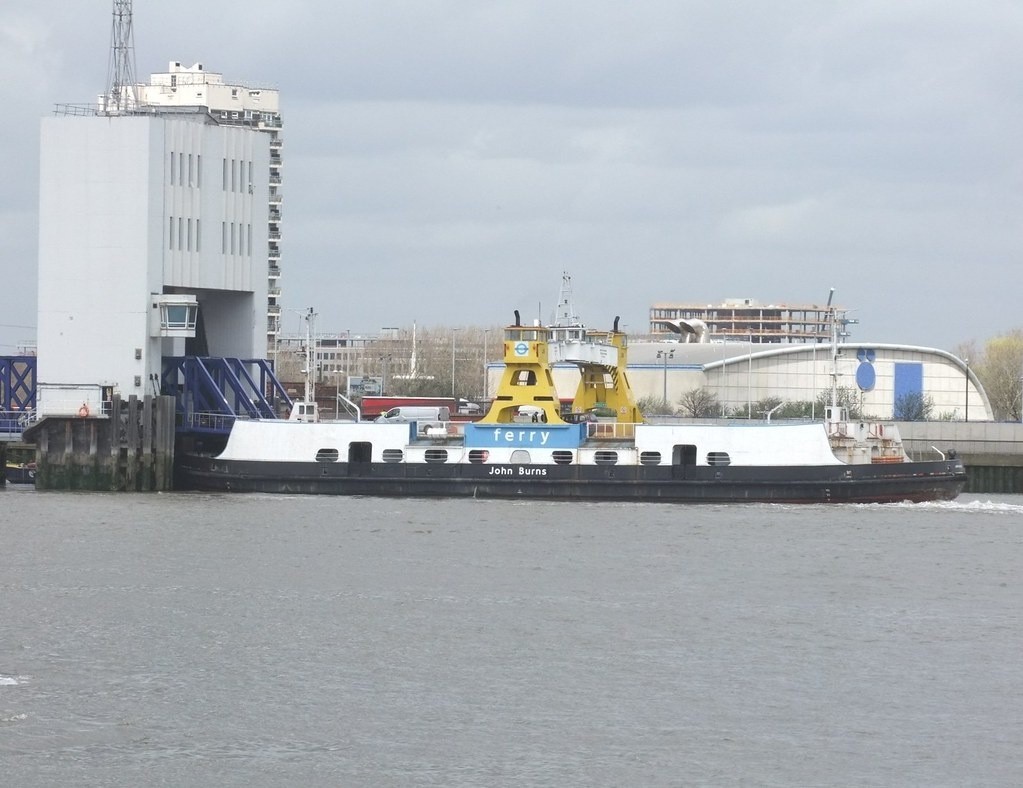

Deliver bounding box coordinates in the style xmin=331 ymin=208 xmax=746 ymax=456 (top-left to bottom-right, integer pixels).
xmin=374 ymin=407 xmax=450 ymax=437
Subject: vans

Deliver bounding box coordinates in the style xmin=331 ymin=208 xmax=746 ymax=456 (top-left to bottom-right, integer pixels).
xmin=586 ymin=407 xmax=618 ymax=418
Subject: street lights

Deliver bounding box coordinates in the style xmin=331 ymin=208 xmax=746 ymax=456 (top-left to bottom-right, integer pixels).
xmin=656 ymin=348 xmax=675 ymax=415
xmin=452 ymin=327 xmax=459 ymax=397
xmin=483 ymin=329 xmax=492 ymax=416
xmin=345 ymin=328 xmax=351 ymax=401
xmin=812 ymin=332 xmax=816 ymax=423
xmin=722 ymin=328 xmax=728 ymax=418
xmin=748 ymin=327 xmax=753 ymax=420
xmin=413 ymin=318 xmax=418 ymax=377
xmin=965 ymin=358 xmax=969 ymax=423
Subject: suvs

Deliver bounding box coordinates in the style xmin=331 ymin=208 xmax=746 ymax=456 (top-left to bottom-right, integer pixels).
xmin=559 ymin=412 xmax=598 ymax=437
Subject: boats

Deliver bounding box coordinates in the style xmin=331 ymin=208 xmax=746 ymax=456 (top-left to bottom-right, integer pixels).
xmin=180 ymin=308 xmax=970 ymax=504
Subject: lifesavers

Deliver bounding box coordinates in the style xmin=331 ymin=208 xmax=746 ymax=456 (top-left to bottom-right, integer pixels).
xmin=79 ymin=406 xmax=89 ymax=417
